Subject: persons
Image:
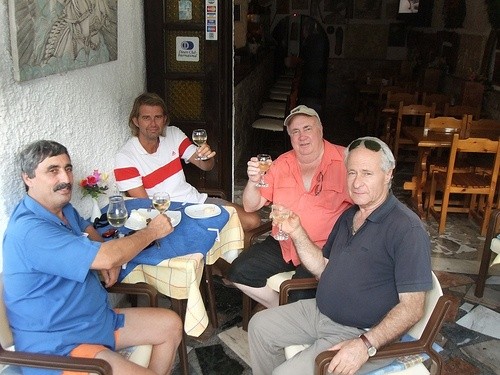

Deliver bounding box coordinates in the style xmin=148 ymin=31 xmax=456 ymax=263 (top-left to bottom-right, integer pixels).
xmin=226 ymin=104 xmax=355 ymax=310
xmin=112 ymin=92 xmax=261 ymax=249
xmin=2 ymin=140 xmax=184 ymax=375
xmin=246 ymin=136 xmax=434 ymax=375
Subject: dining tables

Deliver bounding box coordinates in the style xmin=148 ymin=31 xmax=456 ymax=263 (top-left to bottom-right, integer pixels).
xmin=86 ymin=197 xmax=235 ymax=375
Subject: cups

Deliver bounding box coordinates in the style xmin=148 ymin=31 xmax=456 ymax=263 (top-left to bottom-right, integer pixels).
xmin=106 ymin=195 xmax=129 ymax=227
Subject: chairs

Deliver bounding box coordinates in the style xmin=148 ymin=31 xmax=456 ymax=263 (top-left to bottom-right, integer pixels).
xmin=352 ymin=72 xmax=500 ymax=237
xmin=197 ymin=187 xmax=230 ymax=201
xmin=279 ymin=270 xmax=460 ymax=375
xmin=242 ymin=221 xmax=273 ymax=331
xmin=0 ymin=280 xmax=159 ymax=375
xmin=250 ymin=55 xmax=304 ymax=157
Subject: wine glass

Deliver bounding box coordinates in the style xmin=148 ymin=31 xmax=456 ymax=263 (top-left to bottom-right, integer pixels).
xmin=271 ymin=204 xmax=289 ymax=241
xmin=256 ymin=154 xmax=271 ymax=188
xmin=152 ymin=191 xmax=171 ymax=214
xmin=193 ymin=129 xmax=207 ymax=160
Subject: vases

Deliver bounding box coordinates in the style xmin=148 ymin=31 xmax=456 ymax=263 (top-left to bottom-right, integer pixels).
xmin=90 ymin=198 xmax=103 ymax=222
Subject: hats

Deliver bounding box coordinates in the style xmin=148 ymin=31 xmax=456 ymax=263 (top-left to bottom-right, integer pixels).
xmin=284 ymin=104 xmax=321 ymax=126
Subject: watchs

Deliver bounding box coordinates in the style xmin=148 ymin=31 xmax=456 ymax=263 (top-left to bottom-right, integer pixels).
xmin=358 ymin=334 xmax=377 ymax=357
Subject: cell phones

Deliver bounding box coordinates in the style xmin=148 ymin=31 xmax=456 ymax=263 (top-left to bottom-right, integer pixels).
xmin=103 ymin=229 xmax=118 ymax=238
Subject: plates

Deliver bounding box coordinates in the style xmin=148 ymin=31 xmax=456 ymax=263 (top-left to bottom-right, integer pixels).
xmin=184 ymin=204 xmax=221 ymax=218
xmin=124 ymin=208 xmax=182 ymax=231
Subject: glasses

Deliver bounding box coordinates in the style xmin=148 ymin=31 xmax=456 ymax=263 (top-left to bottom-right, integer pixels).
xmin=348 ymin=139 xmax=388 ymax=159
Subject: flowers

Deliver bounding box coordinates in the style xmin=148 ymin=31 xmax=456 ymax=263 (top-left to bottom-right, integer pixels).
xmin=80 ymin=169 xmax=110 ymax=201
